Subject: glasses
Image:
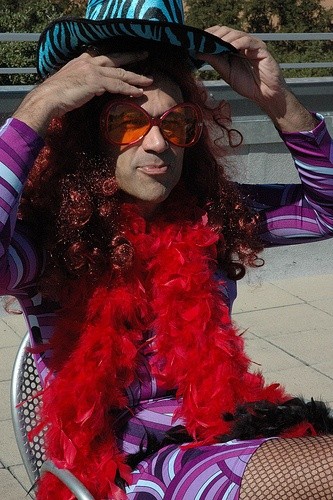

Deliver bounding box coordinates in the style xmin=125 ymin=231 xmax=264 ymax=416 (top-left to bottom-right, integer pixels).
xmin=102 ymin=97 xmax=203 ymax=147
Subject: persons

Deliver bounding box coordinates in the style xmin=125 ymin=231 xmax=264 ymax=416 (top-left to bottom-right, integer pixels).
xmin=1 ymin=0 xmax=333 ymax=500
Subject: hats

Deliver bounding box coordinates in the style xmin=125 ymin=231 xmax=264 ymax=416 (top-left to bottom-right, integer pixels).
xmin=37 ymin=0 xmax=238 ymax=79
